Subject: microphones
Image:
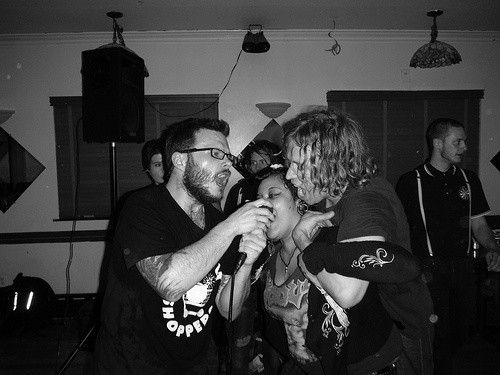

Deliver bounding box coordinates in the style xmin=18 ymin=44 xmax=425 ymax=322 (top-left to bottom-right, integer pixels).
xmin=235 ymin=205 xmax=273 ymax=267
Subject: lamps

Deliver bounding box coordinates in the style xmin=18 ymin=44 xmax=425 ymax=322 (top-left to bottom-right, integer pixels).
xmin=410 ymin=9 xmax=463 ymax=68
xmin=256 ymin=103 xmax=290 ymax=118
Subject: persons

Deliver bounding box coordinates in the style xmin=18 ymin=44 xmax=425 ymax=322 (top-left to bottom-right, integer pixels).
xmin=395 ymin=118 xmax=500 ymax=375
xmin=141 ymin=138 xmax=164 ymax=185
xmin=252 ymin=164 xmax=351 ymax=375
xmin=92 ymin=118 xmax=277 ymax=375
xmin=280 ymin=105 xmax=434 ymax=375
xmin=223 ymin=140 xmax=285 ymax=375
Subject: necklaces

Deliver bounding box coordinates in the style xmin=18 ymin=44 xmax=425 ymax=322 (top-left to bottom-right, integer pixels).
xmin=279 ymin=247 xmax=297 ymax=273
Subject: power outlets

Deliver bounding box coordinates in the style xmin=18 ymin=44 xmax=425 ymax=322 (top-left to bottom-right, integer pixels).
xmin=402 ymin=69 xmax=410 ymax=81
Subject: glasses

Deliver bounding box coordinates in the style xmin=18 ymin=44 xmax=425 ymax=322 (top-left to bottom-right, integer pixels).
xmin=183 ymin=148 xmax=233 ymax=161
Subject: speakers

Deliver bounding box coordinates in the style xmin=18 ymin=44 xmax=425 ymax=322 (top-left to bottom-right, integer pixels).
xmin=0 ymin=125 xmax=45 ymax=213
xmin=81 ymin=47 xmax=144 ymax=144
xmin=10 ymin=277 xmax=57 ymax=331
